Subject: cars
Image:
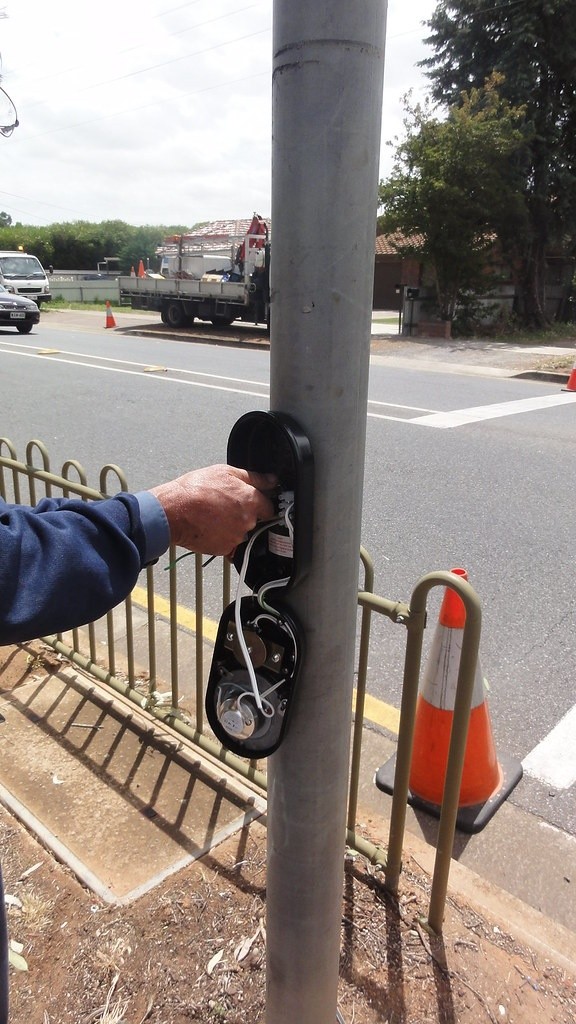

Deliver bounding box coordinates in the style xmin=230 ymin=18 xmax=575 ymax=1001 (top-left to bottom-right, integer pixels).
xmin=0 ymin=284 xmax=40 ymax=335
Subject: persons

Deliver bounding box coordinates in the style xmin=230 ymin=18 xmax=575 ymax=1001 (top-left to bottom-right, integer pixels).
xmin=1 ymin=465 xmax=275 ymax=1023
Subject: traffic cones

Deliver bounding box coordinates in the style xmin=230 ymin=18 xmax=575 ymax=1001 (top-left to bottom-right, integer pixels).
xmin=130 ymin=266 xmax=136 ymax=277
xmin=137 ymin=260 xmax=145 ymax=277
xmin=561 ymin=361 xmax=576 ymax=392
xmin=375 ymin=569 xmax=523 ymax=834
xmin=103 ymin=301 xmax=119 ymax=329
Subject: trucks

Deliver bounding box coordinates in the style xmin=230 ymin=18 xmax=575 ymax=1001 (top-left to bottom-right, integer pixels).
xmin=0 ymin=251 xmax=53 ymax=310
xmin=118 ymin=212 xmax=270 ymax=335
